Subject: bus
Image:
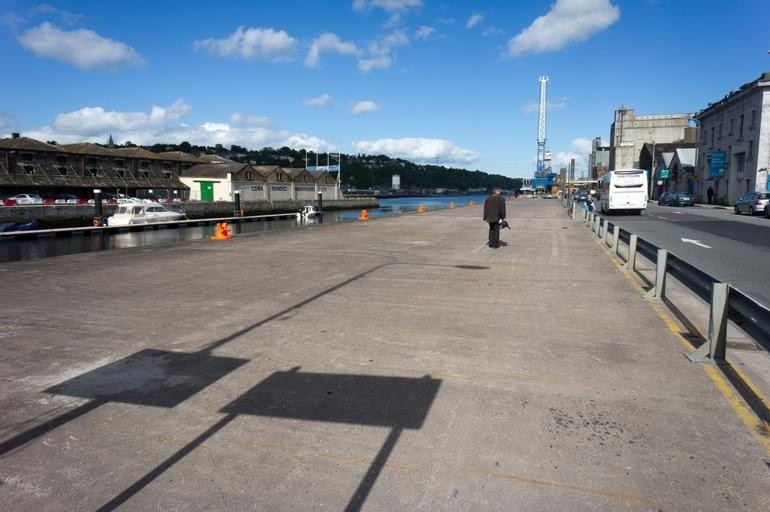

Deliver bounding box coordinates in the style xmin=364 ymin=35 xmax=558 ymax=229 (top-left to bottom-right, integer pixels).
xmin=596 ymin=169 xmax=649 ymax=215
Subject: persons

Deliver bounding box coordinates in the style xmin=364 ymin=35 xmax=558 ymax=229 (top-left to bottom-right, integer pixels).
xmin=584 ymin=193 xmax=595 ymax=222
xmin=482 ymin=187 xmax=506 ymax=248
xmin=707 ymin=186 xmax=713 ymax=202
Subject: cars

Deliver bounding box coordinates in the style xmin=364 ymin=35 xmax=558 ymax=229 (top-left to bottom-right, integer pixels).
xmin=764 ymin=200 xmax=770 ymax=219
xmin=5 ymin=193 xmax=185 ymax=206
xmin=542 ymin=193 xmax=556 ymax=199
xmin=565 ymin=182 xmax=596 ymax=203
xmin=734 ymin=190 xmax=770 ymax=216
xmin=657 ymin=192 xmax=696 ymax=208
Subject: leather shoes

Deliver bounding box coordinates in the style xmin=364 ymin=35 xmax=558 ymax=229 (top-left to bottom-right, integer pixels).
xmin=489 ymin=243 xmax=500 ymax=248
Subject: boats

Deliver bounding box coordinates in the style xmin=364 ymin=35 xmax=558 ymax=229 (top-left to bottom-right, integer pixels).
xmin=107 ymin=196 xmax=191 ymax=226
xmin=296 ymin=205 xmax=325 ymax=219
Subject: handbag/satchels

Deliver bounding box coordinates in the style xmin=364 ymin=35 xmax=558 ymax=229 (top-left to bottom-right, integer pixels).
xmin=500 ymin=219 xmax=508 ymax=229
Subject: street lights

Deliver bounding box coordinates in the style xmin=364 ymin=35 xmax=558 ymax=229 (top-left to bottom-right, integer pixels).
xmin=636 ymin=137 xmax=654 ymax=199
